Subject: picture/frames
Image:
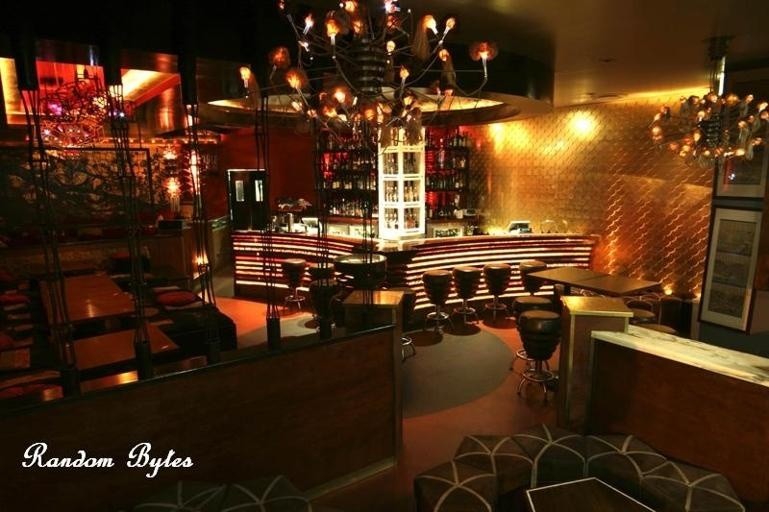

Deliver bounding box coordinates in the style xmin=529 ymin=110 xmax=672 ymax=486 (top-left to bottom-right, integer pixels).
xmin=711 ymin=66 xmax=769 ymax=200
xmin=697 ymin=205 xmax=763 ymax=336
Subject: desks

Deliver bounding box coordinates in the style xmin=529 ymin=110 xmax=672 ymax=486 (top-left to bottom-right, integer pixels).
xmin=0 ymin=250 xmax=236 ymax=412
xmin=316 ymin=253 xmax=393 ymax=322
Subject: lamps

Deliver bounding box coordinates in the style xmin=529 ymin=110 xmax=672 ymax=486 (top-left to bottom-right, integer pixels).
xmin=270 ymin=1 xmax=499 ymax=175
xmin=643 ymin=33 xmax=768 ymax=174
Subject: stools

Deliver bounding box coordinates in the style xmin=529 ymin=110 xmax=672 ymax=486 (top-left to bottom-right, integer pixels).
xmin=132 ymin=463 xmax=313 ymax=512
xmin=415 ymin=422 xmax=747 ymax=512
xmin=280 ymin=257 xmax=696 ymax=404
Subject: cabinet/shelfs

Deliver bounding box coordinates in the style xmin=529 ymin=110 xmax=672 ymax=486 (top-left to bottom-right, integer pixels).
xmin=315 ymin=127 xmax=470 ymax=225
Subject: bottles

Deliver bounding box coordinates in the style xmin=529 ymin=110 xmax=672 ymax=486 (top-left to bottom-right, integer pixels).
xmin=318 ymin=132 xmax=469 ymax=231
